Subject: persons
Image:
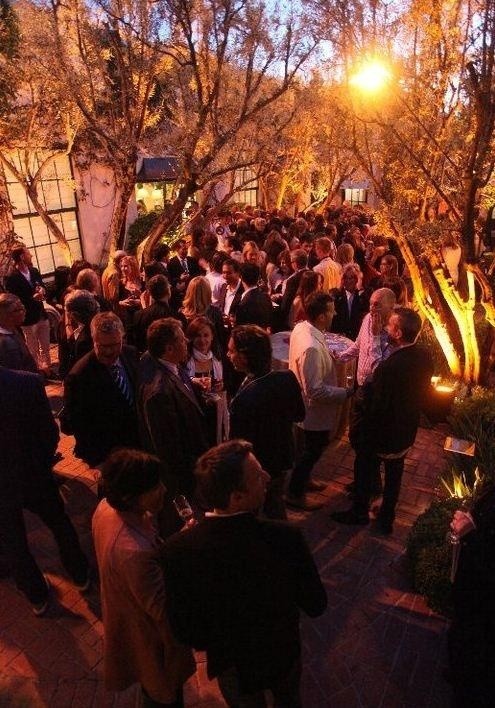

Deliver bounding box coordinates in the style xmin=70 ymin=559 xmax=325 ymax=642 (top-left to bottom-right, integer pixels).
xmin=93 ymin=448 xmax=180 ymax=708
xmin=1 ymin=204 xmax=469 ymax=617
xmin=445 ymin=490 xmax=495 ymax=708
xmin=195 ymin=438 xmax=328 ymax=708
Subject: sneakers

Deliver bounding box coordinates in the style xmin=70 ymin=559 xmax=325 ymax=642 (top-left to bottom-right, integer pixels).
xmin=70 ymin=570 xmax=91 ymax=594
xmin=287 ymin=478 xmax=395 ymax=537
xmin=32 ymin=575 xmax=52 ymax=614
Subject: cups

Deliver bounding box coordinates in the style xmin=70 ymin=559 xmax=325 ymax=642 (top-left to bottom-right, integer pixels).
xmin=321 ymin=330 xmax=352 ymax=360
xmin=223 ymin=312 xmax=237 ymax=327
xmin=127 ymin=294 xmax=136 ymax=306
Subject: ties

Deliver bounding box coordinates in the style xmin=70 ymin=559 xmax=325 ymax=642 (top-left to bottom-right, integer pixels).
xmin=182 ymin=260 xmax=191 ymax=278
xmin=112 ymin=366 xmax=133 ymax=406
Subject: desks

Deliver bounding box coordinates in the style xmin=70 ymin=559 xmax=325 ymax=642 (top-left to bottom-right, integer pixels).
xmin=271 ymin=330 xmax=359 ymax=440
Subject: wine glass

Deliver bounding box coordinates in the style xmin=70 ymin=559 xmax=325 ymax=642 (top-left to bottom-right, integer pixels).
xmin=200 ymin=371 xmax=224 ymax=402
xmin=173 ymin=495 xmax=195 ymax=528
xmin=343 ymin=367 xmax=372 ymax=390
